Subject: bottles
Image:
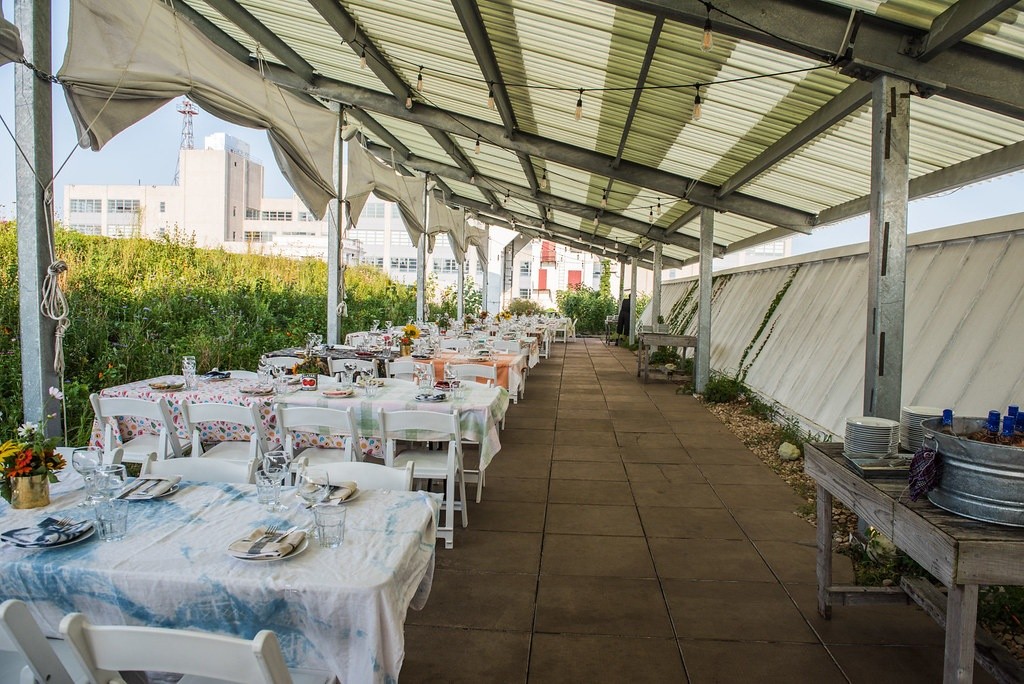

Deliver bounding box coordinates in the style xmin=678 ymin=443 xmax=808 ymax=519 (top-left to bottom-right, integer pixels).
xmin=941 ymin=405 xmax=1024 ymax=448
xmin=301 ymin=351 xmax=318 ymax=391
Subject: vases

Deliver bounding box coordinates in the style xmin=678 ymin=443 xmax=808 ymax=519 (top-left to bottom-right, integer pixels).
xmin=10 ymin=473 xmax=49 ymax=509
xmin=400 ymin=345 xmax=411 ymax=357
xmin=301 ymin=373 xmax=317 ymax=390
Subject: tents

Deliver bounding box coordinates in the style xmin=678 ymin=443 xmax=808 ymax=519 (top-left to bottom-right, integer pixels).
xmin=0 ymin=0 xmax=1024 ymax=547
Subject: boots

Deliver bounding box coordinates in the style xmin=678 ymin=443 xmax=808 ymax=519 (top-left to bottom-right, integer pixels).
xmin=615 ymin=339 xmax=618 ymax=346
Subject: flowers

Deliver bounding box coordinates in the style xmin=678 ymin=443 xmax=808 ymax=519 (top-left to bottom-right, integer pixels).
xmin=291 ymin=356 xmax=323 ymax=375
xmin=395 ymin=323 xmax=421 ymax=353
xmin=0 ymin=386 xmax=67 ymax=503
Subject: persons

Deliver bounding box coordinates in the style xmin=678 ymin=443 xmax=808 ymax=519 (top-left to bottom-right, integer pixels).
xmin=614 ymin=294 xmax=630 ymax=346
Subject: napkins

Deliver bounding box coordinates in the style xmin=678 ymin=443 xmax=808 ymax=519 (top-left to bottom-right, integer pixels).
xmin=415 ymin=394 xmax=445 ymax=401
xmin=355 ymin=379 xmax=384 ymax=387
xmin=149 ymin=382 xmax=185 ymax=389
xmin=433 ymin=381 xmax=460 ymax=389
xmin=228 ymin=527 xmax=307 ymax=560
xmin=295 ymin=479 xmax=357 ymax=505
xmin=201 ymin=370 xmax=231 ymax=380
xmin=0 ymin=517 xmax=94 ymax=548
xmin=322 ymin=387 xmax=353 ymax=396
xmin=116 ymin=474 xmax=182 ymax=499
xmin=238 ymin=385 xmax=273 ymax=394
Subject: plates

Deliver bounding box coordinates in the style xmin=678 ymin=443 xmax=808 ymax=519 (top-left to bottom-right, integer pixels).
xmin=226 ymin=530 xmax=309 ymax=564
xmin=249 ymin=388 xmax=276 ymax=396
xmin=321 ymin=391 xmax=355 ymax=398
xmin=160 ymin=483 xmax=179 ymax=496
xmin=10 ymin=526 xmax=95 ymax=552
xmin=156 ymin=387 xmax=185 ymax=392
xmin=844 ymin=405 xmax=953 ymax=455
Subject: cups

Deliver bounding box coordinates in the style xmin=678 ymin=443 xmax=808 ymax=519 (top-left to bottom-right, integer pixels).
xmin=360 ymin=313 xmax=565 ymax=361
xmin=319 ymin=344 xmax=326 ymax=356
xmin=182 ymin=355 xmax=201 ymax=390
xmin=256 ymin=357 xmax=290 ymax=401
xmin=366 ymin=379 xmax=378 ymax=398
xmin=413 ymin=362 xmax=466 ymax=401
xmin=255 ymin=468 xmax=282 ymax=508
xmin=314 ymin=503 xmax=346 ymax=551
xmin=89 ymin=498 xmax=129 ymax=542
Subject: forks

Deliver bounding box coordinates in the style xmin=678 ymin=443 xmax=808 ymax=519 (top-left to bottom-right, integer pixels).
xmin=254 ymin=524 xmax=278 ymax=544
xmin=54 ymin=516 xmax=73 ymax=527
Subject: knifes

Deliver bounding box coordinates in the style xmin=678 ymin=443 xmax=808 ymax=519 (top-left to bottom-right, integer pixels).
xmin=116 ymin=479 xmax=147 ymax=499
xmin=272 ymin=525 xmax=298 ymax=543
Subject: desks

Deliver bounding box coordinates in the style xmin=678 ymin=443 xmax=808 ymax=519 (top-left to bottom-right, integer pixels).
xmin=803 ymin=442 xmax=1024 ymax=684
xmin=0 ymin=471 xmax=445 ymax=684
xmin=260 ymin=346 xmax=521 ymax=430
xmin=605 ymin=319 xmax=627 ymax=346
xmin=87 ymin=375 xmax=510 ymax=499
xmin=637 ymin=332 xmax=697 ymax=384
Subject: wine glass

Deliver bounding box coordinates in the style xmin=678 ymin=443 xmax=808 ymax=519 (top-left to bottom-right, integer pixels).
xmin=73 ymin=446 xmax=103 ymax=509
xmin=307 ymin=332 xmax=323 ymax=346
xmin=361 ymin=367 xmax=374 ymax=395
xmin=262 ymin=450 xmax=292 ymax=513
xmin=344 ymin=361 xmax=358 ymax=387
xmin=298 ymin=468 xmax=330 ymax=538
xmin=95 ymin=464 xmax=128 ymax=508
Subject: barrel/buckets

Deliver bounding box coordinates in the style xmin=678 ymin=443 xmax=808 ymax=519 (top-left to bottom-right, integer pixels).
xmin=919 ymin=416 xmax=1024 ymax=527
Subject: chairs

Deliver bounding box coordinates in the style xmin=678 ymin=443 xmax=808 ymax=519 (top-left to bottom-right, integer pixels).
xmin=0 ymin=314 xmax=578 ymax=684
